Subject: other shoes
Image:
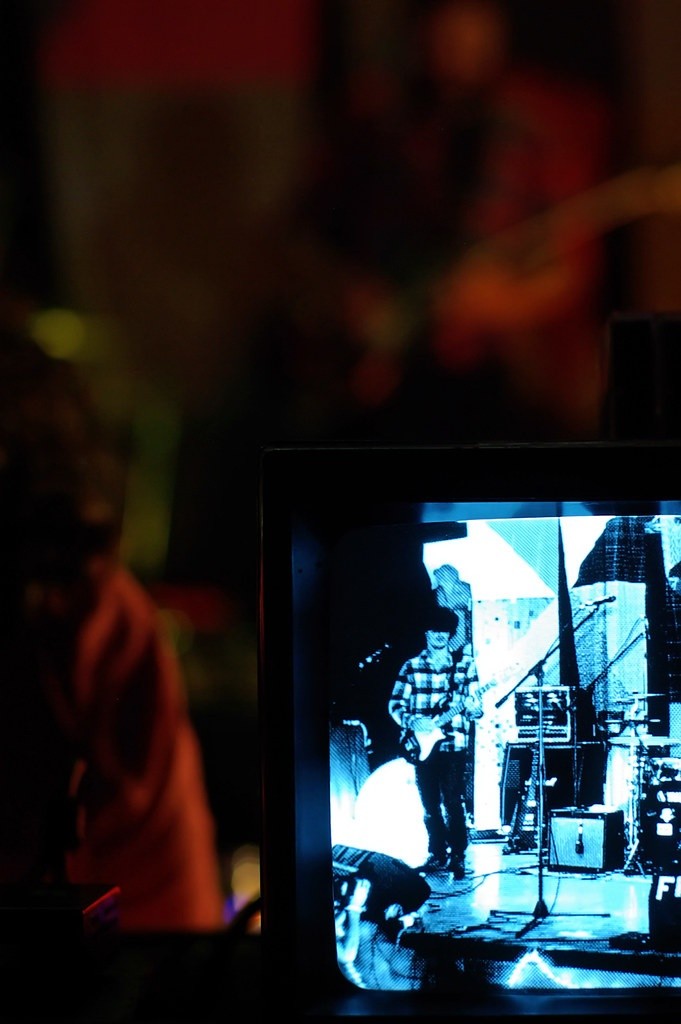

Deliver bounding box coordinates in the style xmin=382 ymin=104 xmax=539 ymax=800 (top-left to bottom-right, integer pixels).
xmin=425 ymin=849 xmax=448 ymax=871
xmin=451 ymin=851 xmax=466 ymax=878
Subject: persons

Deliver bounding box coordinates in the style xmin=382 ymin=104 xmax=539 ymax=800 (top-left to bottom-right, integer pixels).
xmin=0 ymin=321 xmax=225 ymax=936
xmin=388 ymin=607 xmax=484 ymax=879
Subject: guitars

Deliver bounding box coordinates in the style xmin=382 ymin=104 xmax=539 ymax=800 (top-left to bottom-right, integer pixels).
xmin=398 ymin=661 xmax=521 ymax=767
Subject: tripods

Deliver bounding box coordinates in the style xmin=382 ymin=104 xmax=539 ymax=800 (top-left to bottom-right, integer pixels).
xmin=488 ymin=606 xmax=611 ymax=939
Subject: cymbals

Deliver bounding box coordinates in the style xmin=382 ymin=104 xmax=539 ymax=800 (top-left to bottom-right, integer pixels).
xmin=608 ymin=734 xmax=681 ymax=747
xmin=603 ymin=718 xmax=662 ymax=724
xmin=611 ymin=692 xmax=665 ymax=705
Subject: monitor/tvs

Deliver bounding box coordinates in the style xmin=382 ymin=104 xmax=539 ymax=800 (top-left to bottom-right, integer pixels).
xmin=256 ymin=441 xmax=681 ymax=1023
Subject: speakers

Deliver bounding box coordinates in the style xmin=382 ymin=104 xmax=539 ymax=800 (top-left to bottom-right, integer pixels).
xmin=549 ymin=807 xmax=625 ymax=874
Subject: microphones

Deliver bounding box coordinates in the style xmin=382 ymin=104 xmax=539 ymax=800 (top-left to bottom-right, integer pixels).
xmin=575 ymin=824 xmax=584 ymax=853
xmin=585 ymin=595 xmax=616 ymax=606
xmin=629 ymin=721 xmax=647 ymax=753
